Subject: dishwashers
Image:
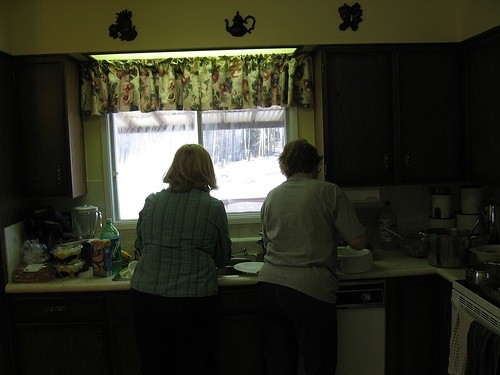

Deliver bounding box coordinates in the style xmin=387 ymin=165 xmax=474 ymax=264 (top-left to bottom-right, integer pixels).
xmin=297 ymin=280 xmax=387 ymax=375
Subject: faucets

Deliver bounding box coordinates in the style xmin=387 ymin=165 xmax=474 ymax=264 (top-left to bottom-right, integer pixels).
xmin=231 ymin=247 xmax=248 ymax=257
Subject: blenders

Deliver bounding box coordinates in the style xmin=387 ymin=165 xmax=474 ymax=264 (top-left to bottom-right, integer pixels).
xmin=70 ymin=206 xmax=101 ymax=271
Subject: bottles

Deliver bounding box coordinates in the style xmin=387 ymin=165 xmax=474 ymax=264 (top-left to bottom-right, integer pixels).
xmin=378 ymin=202 xmax=395 ymax=249
xmin=101 ymin=217 xmax=123 ymax=271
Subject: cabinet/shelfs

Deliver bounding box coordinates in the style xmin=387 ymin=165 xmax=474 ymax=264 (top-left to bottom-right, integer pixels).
xmin=463 ymin=24 xmax=500 ymax=186
xmin=13 ymin=54 xmax=88 ymax=201
xmin=381 ymin=274 xmax=453 ymax=375
xmin=310 ymin=42 xmax=465 ymax=188
xmin=0 ymin=286 xmax=261 ymax=375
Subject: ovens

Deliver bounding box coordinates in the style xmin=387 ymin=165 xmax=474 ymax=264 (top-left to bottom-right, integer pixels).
xmin=450 ymin=289 xmax=500 ymax=337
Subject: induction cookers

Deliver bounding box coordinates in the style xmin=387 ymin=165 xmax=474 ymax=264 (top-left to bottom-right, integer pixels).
xmin=453 ymin=275 xmax=500 ymax=318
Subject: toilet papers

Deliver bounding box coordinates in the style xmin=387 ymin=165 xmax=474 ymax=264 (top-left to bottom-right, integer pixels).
xmin=432 ymin=193 xmax=453 ymax=219
xmin=459 ymin=186 xmax=485 ymax=214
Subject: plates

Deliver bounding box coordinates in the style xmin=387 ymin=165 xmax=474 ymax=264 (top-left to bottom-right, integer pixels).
xmin=128 ymin=260 xmax=138 ymax=274
xmin=235 ymin=261 xmax=263 ymax=274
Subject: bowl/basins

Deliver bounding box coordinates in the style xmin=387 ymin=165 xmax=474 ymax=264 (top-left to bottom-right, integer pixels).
xmin=52 ymin=243 xmax=84 ymax=278
xmin=398 ymin=232 xmax=434 ymax=258
xmin=470 ymin=244 xmax=500 ymax=262
xmin=337 ymin=246 xmax=372 ymax=273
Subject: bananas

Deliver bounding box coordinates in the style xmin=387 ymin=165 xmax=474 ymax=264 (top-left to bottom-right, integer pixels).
xmin=121 ymin=251 xmax=131 ymax=261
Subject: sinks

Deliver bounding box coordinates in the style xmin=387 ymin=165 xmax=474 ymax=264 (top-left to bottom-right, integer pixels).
xmin=215 ymin=255 xmax=258 ymax=278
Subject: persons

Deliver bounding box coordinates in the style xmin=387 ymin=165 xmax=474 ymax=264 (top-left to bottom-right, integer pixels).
xmin=130 ymin=144 xmax=231 ymax=375
xmin=256 ymin=141 xmax=367 ymax=375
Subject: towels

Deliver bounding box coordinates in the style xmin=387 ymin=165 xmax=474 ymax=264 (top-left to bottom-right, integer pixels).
xmin=464 ymin=320 xmax=500 ymax=375
xmin=447 ymin=305 xmax=476 ymax=375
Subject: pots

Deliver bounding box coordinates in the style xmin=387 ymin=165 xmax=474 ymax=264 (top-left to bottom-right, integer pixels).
xmin=465 ymin=264 xmax=494 ymax=288
xmin=419 ymin=227 xmax=481 ymax=269
